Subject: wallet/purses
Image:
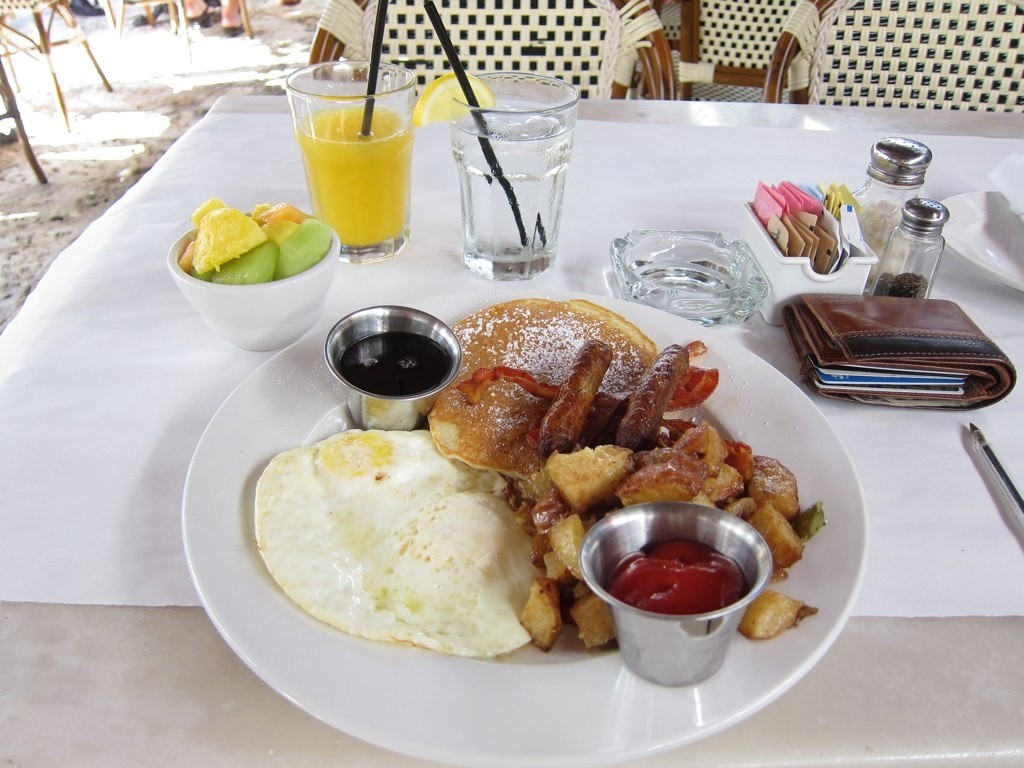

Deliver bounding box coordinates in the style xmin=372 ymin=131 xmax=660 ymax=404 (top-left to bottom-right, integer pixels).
xmin=781 ymin=293 xmax=1017 ymax=411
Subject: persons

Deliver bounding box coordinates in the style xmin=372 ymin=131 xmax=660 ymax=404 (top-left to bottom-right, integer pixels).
xmin=183 ymin=0 xmax=245 ymax=36
xmin=70 ymin=0 xmax=106 ymax=16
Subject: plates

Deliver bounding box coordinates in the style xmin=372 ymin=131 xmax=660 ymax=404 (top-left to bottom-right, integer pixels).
xmin=936 ymin=190 xmax=1023 ymax=293
xmin=180 ymin=289 xmax=869 ymax=768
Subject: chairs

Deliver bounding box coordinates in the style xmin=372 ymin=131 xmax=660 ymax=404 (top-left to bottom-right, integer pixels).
xmin=636 ymin=0 xmax=801 ymax=101
xmin=307 ymin=0 xmax=678 ymax=104
xmin=760 ymin=0 xmax=1023 ymax=114
xmin=0 ymin=1 xmax=256 ymax=188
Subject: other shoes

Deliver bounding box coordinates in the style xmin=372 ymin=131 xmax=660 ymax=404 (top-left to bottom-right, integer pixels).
xmin=68 ymin=0 xmax=105 ymax=16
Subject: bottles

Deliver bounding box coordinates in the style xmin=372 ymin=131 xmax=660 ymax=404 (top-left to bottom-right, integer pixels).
xmin=867 ymin=198 xmax=950 ymax=300
xmin=853 ymin=137 xmax=932 ymax=297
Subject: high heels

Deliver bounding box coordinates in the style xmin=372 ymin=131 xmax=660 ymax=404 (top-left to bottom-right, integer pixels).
xmin=132 ymin=2 xmax=178 ymax=27
xmin=223 ymin=6 xmax=245 ymax=38
xmin=173 ymin=5 xmax=215 ymax=36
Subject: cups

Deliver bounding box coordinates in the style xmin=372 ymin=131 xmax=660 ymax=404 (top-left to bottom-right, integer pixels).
xmin=167 ymin=212 xmax=339 ymax=352
xmin=323 ymin=306 xmax=463 ymax=432
xmin=451 ymin=71 xmax=578 ymax=281
xmin=286 ymin=60 xmax=417 ymax=263
xmin=579 ymin=501 xmax=773 ymax=690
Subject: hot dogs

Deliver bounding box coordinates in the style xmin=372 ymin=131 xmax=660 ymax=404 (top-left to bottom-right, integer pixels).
xmin=178 ymin=170 xmax=842 ymax=691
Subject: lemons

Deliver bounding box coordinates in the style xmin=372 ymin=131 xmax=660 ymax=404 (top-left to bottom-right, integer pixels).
xmin=408 ymin=72 xmax=494 ymax=123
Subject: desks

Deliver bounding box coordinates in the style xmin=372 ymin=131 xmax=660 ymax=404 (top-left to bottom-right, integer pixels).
xmin=0 ymin=91 xmax=1022 ymax=767
